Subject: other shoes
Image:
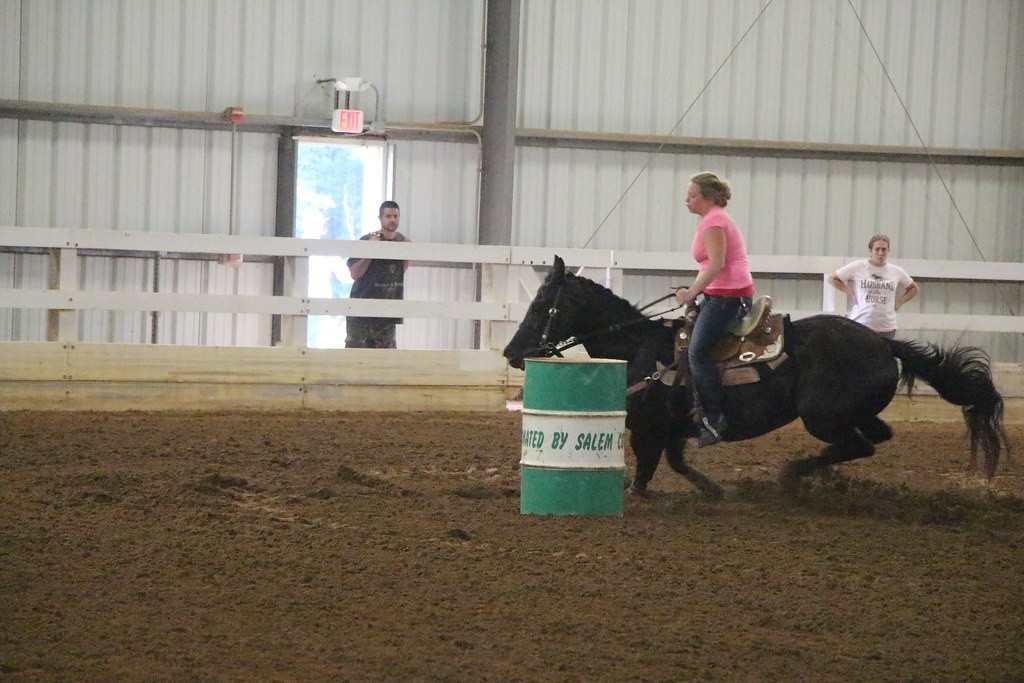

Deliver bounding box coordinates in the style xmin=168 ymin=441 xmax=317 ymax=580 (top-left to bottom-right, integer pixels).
xmin=700 ymin=413 xmax=728 ymax=445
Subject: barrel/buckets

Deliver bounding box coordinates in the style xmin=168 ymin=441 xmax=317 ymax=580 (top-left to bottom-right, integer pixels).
xmin=520 ymin=358 xmax=628 ymax=515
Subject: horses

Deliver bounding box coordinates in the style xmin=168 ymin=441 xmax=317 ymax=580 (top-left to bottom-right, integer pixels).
xmin=503 ymin=254 xmax=1011 ymax=500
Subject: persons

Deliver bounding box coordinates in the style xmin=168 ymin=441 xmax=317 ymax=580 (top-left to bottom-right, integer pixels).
xmin=675 ymin=173 xmax=755 ymax=447
xmin=827 ymin=234 xmax=919 ymax=339
xmin=344 ymin=201 xmax=413 ymax=349
